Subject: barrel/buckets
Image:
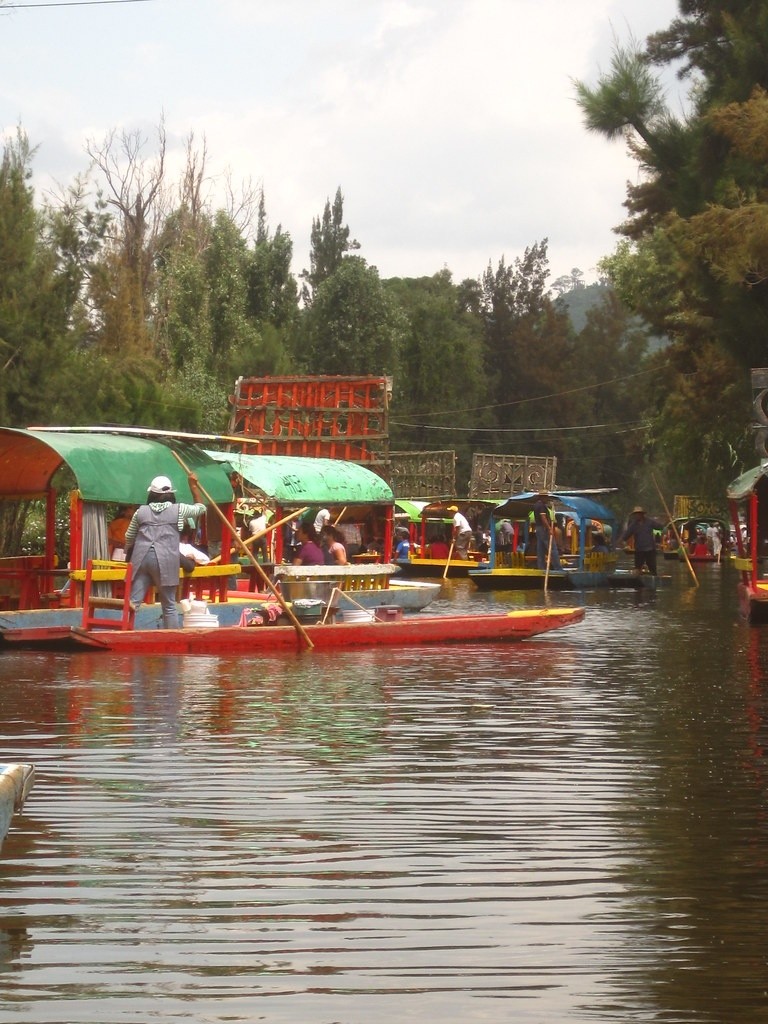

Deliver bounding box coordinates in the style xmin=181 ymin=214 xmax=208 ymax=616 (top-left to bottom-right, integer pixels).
xmin=280 ymin=580 xmax=346 ymax=610
xmin=287 ymin=600 xmax=324 ymax=626
xmin=182 ymin=613 xmax=220 ymax=630
xmin=189 ymin=601 xmax=207 ymax=613
xmin=341 ymin=608 xmax=376 ymax=626
xmin=370 ymin=601 xmax=404 ymax=623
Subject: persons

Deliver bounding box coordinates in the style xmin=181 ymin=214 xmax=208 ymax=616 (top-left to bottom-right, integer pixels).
xmin=739 ymin=525 xmax=747 ymax=553
xmin=619 ymin=505 xmax=669 ymax=575
xmin=313 ymin=507 xmax=335 ymax=532
xmin=321 ymin=526 xmax=347 ymax=565
xmin=707 ymin=524 xmax=712 ymax=543
xmin=713 ymin=522 xmax=723 ymax=565
xmin=393 ymin=520 xmax=409 ymax=535
xmin=446 ymin=505 xmax=472 ymax=561
xmin=472 ymin=516 xmax=612 ymax=558
xmin=292 ymin=522 xmax=325 ymax=566
xmin=394 ymin=532 xmax=417 ymax=560
xmin=122 ymin=473 xmax=206 ymax=630
xmin=427 ymin=535 xmax=449 ymax=559
xmin=532 ymin=489 xmax=566 ymax=572
xmin=100 ymin=504 xmax=139 ymax=598
xmin=340 ymin=517 xmax=360 ymax=563
xmin=168 ymin=521 xmax=209 ymax=572
xmin=248 ymin=509 xmax=269 ymax=565
xmin=281 ymin=508 xmax=301 ymax=558
xmin=680 ymin=536 xmax=711 ymax=557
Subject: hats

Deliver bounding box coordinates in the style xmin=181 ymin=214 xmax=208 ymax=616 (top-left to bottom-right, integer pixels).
xmin=147 ymin=476 xmax=173 ymax=494
xmin=538 ymin=489 xmax=549 ymax=496
xmin=629 ymin=506 xmax=647 ymax=513
xmin=447 ymin=505 xmax=458 ymax=512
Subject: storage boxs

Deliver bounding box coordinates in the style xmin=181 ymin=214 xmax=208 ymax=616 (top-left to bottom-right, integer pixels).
xmin=183 ymin=614 xmax=219 ymax=628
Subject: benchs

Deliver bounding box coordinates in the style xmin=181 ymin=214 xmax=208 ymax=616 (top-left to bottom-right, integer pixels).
xmin=0 ymin=546 xmax=620 ymax=611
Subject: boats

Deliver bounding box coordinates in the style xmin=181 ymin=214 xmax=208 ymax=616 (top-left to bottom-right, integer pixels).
xmin=69 ymin=604 xmax=586 ymax=653
xmin=726 ymin=464 xmax=768 ymax=625
xmin=606 ymin=573 xmax=672 ymax=589
xmin=411 ymin=498 xmax=551 ymax=570
xmin=0 ymin=425 xmax=285 ymax=647
xmin=467 ymin=491 xmax=654 ymax=592
xmin=393 ymin=498 xmax=456 ymax=568
xmin=198 ymin=448 xmax=442 ymax=614
xmin=621 ymin=513 xmax=748 ymax=564
